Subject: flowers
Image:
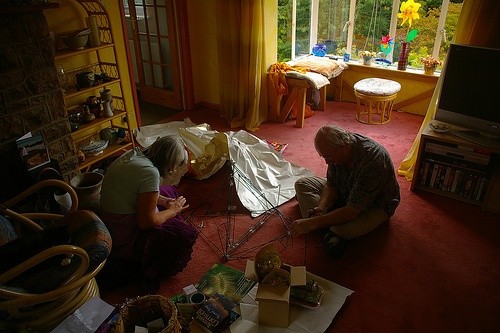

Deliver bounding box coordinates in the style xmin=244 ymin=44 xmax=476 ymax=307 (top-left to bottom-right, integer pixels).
xmin=419 ymin=55 xmax=443 ymax=67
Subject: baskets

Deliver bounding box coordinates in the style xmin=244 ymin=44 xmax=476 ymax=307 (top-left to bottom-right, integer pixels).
xmin=117 ymin=295 xmax=182 ymax=333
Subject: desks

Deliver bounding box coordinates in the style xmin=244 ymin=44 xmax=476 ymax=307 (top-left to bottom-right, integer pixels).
xmin=266 ymin=56 xmax=441 ymax=128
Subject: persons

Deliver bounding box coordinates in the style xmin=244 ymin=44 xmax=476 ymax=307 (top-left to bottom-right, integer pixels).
xmin=100 ymin=135 xmax=197 ymax=293
xmin=288 ymin=125 xmax=401 ymax=260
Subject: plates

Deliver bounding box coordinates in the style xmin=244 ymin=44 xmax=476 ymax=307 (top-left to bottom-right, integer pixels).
xmin=290 ymin=288 xmax=324 ymax=309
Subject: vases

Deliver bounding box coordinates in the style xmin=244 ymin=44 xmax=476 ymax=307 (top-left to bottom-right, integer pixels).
xmin=423 ymin=65 xmax=437 ymax=75
xmin=69 ymin=172 xmax=104 ymax=211
xmin=397 ymin=42 xmax=410 ymax=71
xmin=81 ymin=90 xmax=115 ymax=124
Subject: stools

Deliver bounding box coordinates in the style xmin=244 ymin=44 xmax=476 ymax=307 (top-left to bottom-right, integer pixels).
xmin=354 ymin=77 xmax=401 ymax=125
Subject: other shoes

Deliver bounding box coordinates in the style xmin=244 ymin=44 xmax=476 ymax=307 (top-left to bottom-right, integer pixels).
xmin=320 ymin=229 xmax=330 ymax=241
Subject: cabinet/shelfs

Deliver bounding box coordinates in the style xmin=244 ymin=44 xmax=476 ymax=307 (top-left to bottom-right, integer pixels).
xmin=0 ymin=158 xmax=63 ymax=205
xmin=410 ymin=120 xmax=500 ymax=210
xmin=42 ymin=0 xmax=135 ymax=170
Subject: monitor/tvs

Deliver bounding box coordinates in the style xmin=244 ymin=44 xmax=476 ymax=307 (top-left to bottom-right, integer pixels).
xmin=435 ymin=44 xmax=500 ymax=149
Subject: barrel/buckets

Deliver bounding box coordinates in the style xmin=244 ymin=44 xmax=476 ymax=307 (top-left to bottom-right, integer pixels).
xmin=112 ymin=295 xmax=182 ymax=333
xmin=71 ymin=172 xmax=104 ymax=214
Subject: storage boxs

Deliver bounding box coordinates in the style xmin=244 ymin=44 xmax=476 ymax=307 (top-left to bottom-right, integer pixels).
xmin=244 ymin=259 xmax=306 ymax=328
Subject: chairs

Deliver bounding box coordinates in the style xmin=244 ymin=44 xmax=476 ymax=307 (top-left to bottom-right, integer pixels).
xmin=0 ymin=179 xmax=112 ymax=333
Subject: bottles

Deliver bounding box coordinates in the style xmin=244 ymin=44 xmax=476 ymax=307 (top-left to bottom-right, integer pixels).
xmin=291 ymin=279 xmax=322 ymax=304
xmin=68 ymin=86 xmax=113 ymax=130
xmin=190 ymin=292 xmax=208 ymax=305
xmin=100 ymin=128 xmax=125 ymax=146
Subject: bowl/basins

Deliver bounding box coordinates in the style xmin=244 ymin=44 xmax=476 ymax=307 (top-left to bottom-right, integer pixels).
xmin=80 ymin=139 xmax=109 ymax=156
xmin=74 ymin=71 xmax=95 ymax=87
xmin=65 ymin=34 xmax=89 ymax=50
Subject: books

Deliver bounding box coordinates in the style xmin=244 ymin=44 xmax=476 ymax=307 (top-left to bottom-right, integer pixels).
xmin=193 ymin=292 xmax=238 ymax=333
xmin=416 ymin=160 xmax=489 ymax=203
